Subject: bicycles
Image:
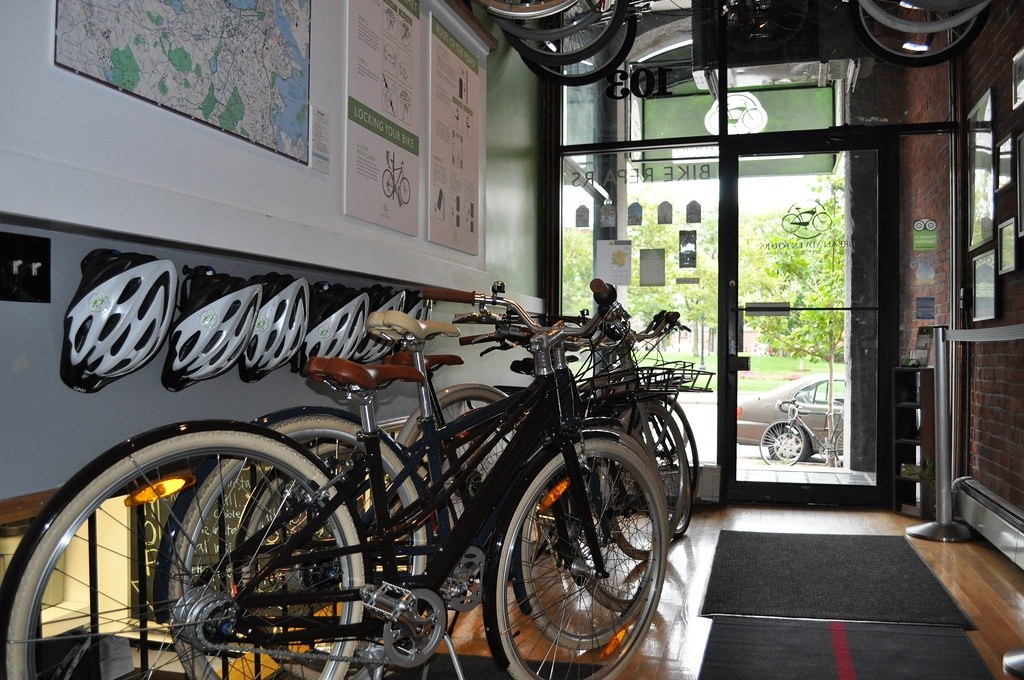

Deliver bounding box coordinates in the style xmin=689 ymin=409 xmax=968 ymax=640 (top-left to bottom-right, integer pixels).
xmin=759 ymin=391 xmax=846 ymax=468
xmin=0 ymin=278 xmax=716 ymax=680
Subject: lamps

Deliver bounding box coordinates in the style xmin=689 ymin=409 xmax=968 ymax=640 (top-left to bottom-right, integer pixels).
xmin=704 ymin=69 xmax=719 ymax=100
xmin=817 ymin=60 xmax=829 ymax=88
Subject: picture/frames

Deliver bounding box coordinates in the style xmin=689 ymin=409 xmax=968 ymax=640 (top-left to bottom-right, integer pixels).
xmin=971 ymin=249 xmax=997 ymax=323
xmin=1016 ymin=131 xmax=1024 ymax=238
xmin=966 ymin=86 xmax=997 ymax=254
xmin=997 ymin=217 xmax=1017 ymax=275
xmin=1011 ymin=46 xmax=1024 ymax=112
xmin=995 ymin=132 xmax=1016 ymax=193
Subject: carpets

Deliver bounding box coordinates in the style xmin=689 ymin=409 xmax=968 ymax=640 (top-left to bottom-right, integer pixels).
xmin=696 ymin=615 xmax=997 ymax=680
xmin=263 ymin=649 xmax=603 ymax=680
xmin=698 ymin=529 xmax=978 ymax=629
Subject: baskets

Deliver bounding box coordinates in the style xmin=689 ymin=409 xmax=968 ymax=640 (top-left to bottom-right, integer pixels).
xmin=575 ymin=360 xmax=717 ymax=410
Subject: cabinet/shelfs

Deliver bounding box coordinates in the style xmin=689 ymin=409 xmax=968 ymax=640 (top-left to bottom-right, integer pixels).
xmin=891 ymin=367 xmax=937 ymax=519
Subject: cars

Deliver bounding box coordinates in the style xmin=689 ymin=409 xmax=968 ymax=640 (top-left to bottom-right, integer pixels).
xmin=737 ymin=371 xmax=845 ymax=462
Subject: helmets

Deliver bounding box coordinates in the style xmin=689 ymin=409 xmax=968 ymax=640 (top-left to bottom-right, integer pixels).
xmin=60 ymin=248 xmax=180 ymax=394
xmin=290 ymin=280 xmax=370 ymax=377
xmin=239 ymin=271 xmax=310 ymax=384
xmin=350 ymin=284 xmax=406 ymax=364
xmin=160 ymin=265 xmax=263 ymax=394
xmin=388 ymin=289 xmax=432 ymax=357
